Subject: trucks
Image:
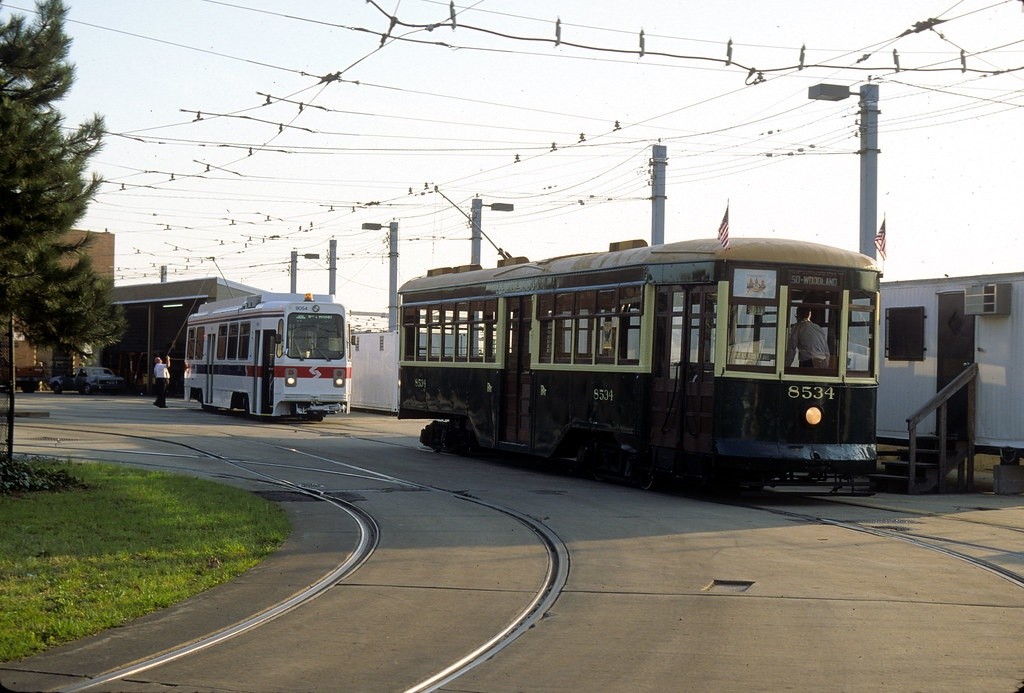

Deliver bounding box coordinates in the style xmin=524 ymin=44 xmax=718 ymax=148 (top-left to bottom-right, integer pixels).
xmin=0 ymin=357 xmax=43 ymax=393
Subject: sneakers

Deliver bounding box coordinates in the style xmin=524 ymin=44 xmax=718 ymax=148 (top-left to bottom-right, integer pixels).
xmin=153 ymin=401 xmax=160 ymax=407
xmin=159 ymin=404 xmax=169 ymax=408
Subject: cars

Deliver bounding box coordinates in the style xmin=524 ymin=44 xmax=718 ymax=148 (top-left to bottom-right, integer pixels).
xmin=75 ymin=366 xmax=124 ymax=395
xmin=49 ymin=375 xmax=76 ymax=394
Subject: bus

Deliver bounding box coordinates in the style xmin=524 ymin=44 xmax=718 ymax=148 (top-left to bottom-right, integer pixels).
xmin=185 ymin=301 xmax=351 ymax=421
xmin=396 ymin=208 xmax=886 ymax=504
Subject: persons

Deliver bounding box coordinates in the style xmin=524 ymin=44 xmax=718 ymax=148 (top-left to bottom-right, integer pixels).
xmin=937 ymin=315 xmax=973 ymax=434
xmin=153 ymin=355 xmax=170 ymax=408
xmin=785 ymin=306 xmax=830 ymax=369
